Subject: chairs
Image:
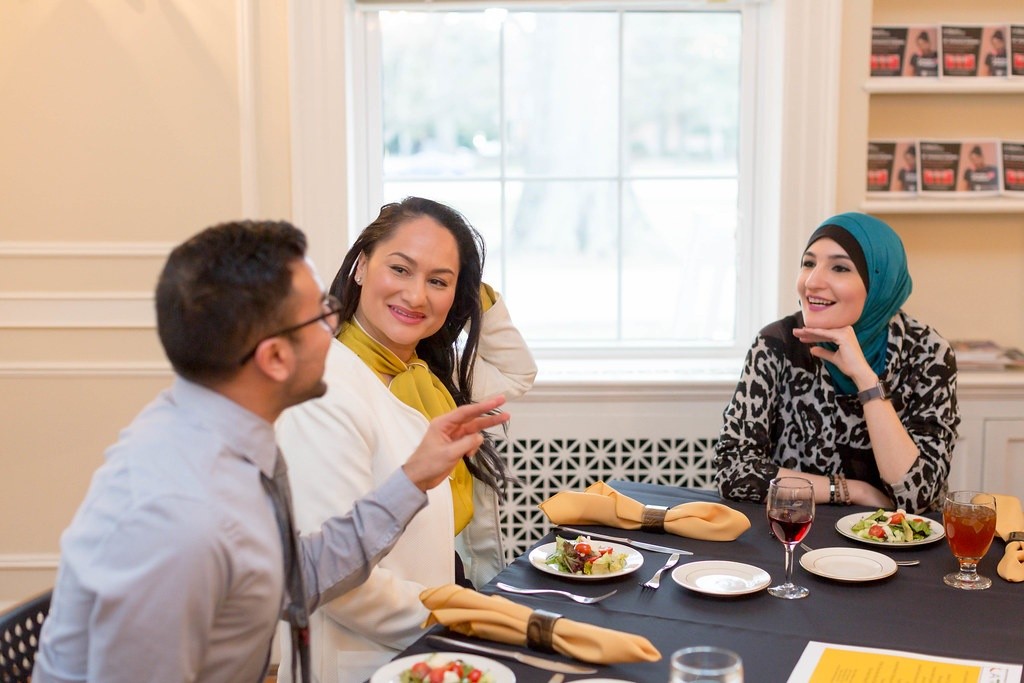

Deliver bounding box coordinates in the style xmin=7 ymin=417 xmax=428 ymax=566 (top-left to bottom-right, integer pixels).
xmin=0 ymin=586 xmax=57 ymax=683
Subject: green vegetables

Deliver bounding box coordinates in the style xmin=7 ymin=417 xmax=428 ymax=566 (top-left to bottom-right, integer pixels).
xmin=398 ymin=660 xmax=474 ymax=683
xmin=851 ymin=509 xmax=932 ymax=543
xmin=544 ymin=534 xmax=625 ymax=576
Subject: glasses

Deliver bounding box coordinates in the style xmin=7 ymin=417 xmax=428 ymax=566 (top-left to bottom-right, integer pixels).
xmin=234 ymin=293 xmax=347 ymax=368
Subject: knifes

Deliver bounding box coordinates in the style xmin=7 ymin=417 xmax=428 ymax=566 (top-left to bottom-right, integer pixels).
xmin=426 ymin=635 xmax=598 ymax=674
xmin=562 ymin=527 xmax=693 ymax=555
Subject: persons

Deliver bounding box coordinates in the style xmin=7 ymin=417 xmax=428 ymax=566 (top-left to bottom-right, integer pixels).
xmin=274 ymin=195 xmax=538 ymax=683
xmin=982 ymin=31 xmax=1006 ymax=75
xmin=892 ymin=146 xmax=917 ymax=191
xmin=958 ymin=145 xmax=997 ymax=190
xmin=714 ymin=212 xmax=962 ymax=515
xmin=31 ymin=219 xmax=510 ymax=683
xmin=907 ymin=32 xmax=938 ymax=75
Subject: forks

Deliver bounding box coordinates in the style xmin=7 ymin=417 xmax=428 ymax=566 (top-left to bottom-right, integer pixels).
xmin=497 ymin=582 xmax=617 ymax=603
xmin=799 ymin=541 xmax=920 ymax=565
xmin=638 ymin=553 xmax=679 ymax=588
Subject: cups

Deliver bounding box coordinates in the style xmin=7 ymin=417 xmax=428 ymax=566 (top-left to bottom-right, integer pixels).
xmin=669 ymin=647 xmax=744 ymax=683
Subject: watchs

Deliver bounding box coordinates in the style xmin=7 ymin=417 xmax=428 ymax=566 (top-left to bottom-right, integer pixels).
xmin=857 ymin=380 xmax=892 ymax=406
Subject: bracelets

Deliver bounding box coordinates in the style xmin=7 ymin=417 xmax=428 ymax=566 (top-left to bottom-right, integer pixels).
xmin=826 ymin=472 xmax=851 ymax=505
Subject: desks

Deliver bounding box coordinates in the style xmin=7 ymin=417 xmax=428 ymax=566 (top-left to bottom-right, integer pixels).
xmin=352 ymin=481 xmax=1024 ymax=683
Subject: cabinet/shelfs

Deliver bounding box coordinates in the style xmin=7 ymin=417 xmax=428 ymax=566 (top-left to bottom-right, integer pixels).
xmin=946 ymin=387 xmax=1024 ymax=503
xmin=863 ymin=75 xmax=1023 ymax=214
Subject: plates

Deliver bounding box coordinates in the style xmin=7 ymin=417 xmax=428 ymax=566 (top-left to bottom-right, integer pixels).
xmin=529 ymin=540 xmax=644 ymax=580
xmin=371 ymin=652 xmax=516 ymax=683
xmin=565 ymin=678 xmax=636 ymax=683
xmin=672 ymin=560 xmax=772 ymax=597
xmin=799 ymin=547 xmax=898 ymax=582
xmin=836 ymin=512 xmax=946 ymax=547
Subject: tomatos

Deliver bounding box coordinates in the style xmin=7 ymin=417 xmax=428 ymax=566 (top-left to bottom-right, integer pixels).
xmin=410 ymin=662 xmax=482 ymax=683
xmin=869 ymin=525 xmax=885 ymax=538
xmin=574 ymin=542 xmax=591 ymax=555
xmin=586 ymin=555 xmax=599 ymax=562
xmin=599 ymin=546 xmax=613 ymax=554
xmin=889 ymin=513 xmax=905 ymax=524
xmin=914 ymin=518 xmax=924 ymax=522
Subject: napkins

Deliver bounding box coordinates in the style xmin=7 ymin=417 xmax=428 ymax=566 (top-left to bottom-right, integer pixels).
xmin=539 ymin=481 xmax=751 ymax=544
xmin=969 ymin=493 xmax=1024 ymax=583
xmin=418 ymin=582 xmax=663 ymax=664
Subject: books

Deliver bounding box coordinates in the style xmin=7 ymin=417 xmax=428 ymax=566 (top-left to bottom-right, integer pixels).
xmin=947 ymin=340 xmax=1024 ymax=373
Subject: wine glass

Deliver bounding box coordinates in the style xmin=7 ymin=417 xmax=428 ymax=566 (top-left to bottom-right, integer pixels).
xmin=943 ymin=492 xmax=997 ymax=590
xmin=767 ymin=477 xmax=815 ymax=599
